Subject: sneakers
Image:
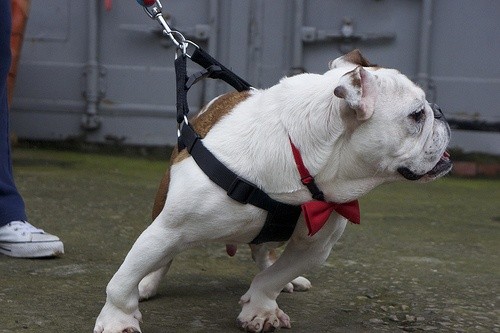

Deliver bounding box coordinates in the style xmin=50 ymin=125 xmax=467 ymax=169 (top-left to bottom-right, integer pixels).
xmin=0 ymin=221 xmax=64 ymax=259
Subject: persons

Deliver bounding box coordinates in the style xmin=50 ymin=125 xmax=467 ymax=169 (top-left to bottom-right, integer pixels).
xmin=0 ymin=0 xmax=63 ymax=258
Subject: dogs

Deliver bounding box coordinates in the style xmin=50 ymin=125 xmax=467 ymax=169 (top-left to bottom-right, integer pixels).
xmin=93 ymin=48 xmax=454 ymax=333
